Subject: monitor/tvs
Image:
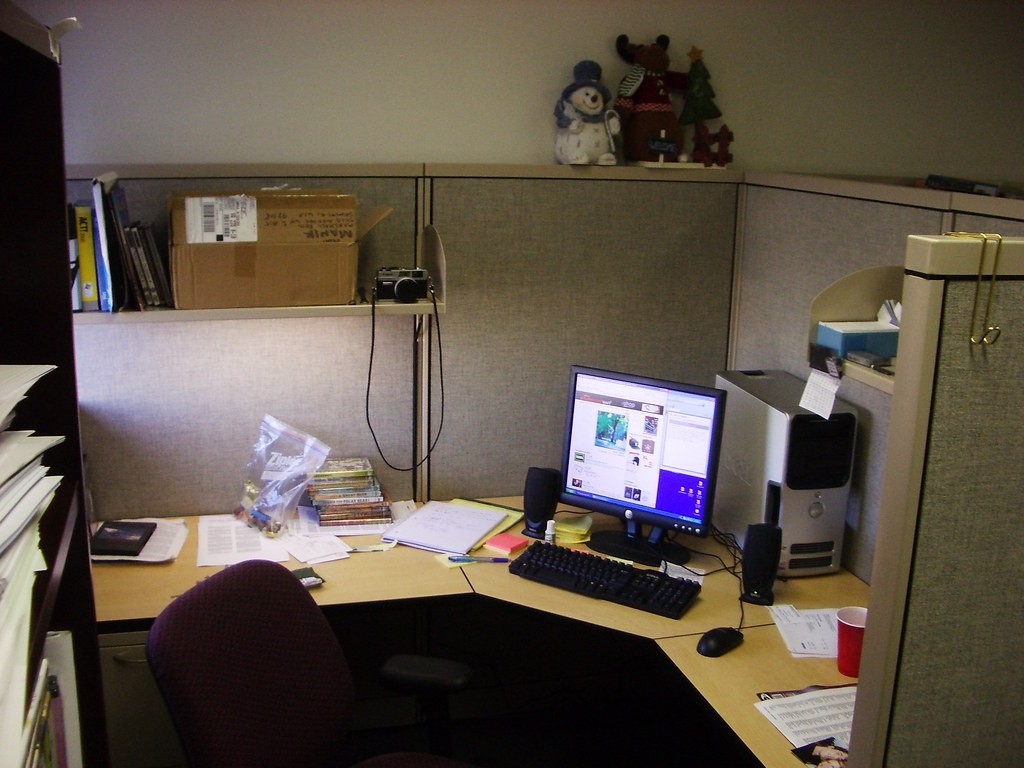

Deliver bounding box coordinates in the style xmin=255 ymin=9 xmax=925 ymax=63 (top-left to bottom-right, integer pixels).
xmin=559 ymin=363 xmax=727 ymax=565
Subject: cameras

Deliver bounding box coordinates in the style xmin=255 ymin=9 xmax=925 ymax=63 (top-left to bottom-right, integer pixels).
xmin=379 ymin=268 xmax=431 ymax=303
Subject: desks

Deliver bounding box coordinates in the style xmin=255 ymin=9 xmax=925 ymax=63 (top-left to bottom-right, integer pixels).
xmin=89 ymin=495 xmax=870 ymax=768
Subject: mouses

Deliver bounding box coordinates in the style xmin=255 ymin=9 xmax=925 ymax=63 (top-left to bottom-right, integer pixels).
xmin=696 ymin=626 xmax=744 ymax=658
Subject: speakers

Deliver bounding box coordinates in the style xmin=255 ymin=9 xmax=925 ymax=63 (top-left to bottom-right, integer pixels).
xmin=521 ymin=467 xmax=563 ymax=540
xmin=737 ymin=524 xmax=782 ymax=607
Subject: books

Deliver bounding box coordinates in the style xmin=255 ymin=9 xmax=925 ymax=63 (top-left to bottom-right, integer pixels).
xmin=303 ymin=454 xmax=394 ymax=527
xmin=92 ymin=519 xmax=157 ymax=556
xmin=67 ymin=170 xmax=172 ymax=311
xmin=382 ymin=499 xmax=509 ymax=555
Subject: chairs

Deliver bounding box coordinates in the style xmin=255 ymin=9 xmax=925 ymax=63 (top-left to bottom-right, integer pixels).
xmin=145 ymin=560 xmax=481 ymax=768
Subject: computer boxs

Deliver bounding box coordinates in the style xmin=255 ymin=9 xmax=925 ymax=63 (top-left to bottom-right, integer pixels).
xmin=711 ymin=370 xmax=860 ymax=577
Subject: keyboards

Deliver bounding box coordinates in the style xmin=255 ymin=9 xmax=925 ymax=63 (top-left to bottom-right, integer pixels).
xmin=507 ymin=540 xmax=702 ymax=620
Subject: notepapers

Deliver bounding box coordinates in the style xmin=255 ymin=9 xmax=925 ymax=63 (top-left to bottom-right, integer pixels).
xmin=555 ymin=515 xmax=594 ymax=544
xmin=482 ymin=532 xmax=530 ymax=557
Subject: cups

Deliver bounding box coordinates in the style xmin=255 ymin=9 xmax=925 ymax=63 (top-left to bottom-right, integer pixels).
xmin=835 ymin=606 xmax=868 ymax=678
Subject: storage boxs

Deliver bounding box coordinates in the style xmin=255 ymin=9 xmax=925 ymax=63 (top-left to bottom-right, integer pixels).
xmin=167 ymin=189 xmax=394 ymax=310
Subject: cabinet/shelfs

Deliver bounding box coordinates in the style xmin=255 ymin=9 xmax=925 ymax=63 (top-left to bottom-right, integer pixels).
xmin=0 ymin=0 xmax=113 ymax=768
xmin=97 ymin=631 xmax=191 ymax=768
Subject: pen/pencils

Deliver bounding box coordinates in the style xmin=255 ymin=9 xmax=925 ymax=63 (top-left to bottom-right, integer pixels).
xmin=447 ymin=555 xmax=511 ymax=564
xmin=347 ymin=548 xmax=380 ymax=553
